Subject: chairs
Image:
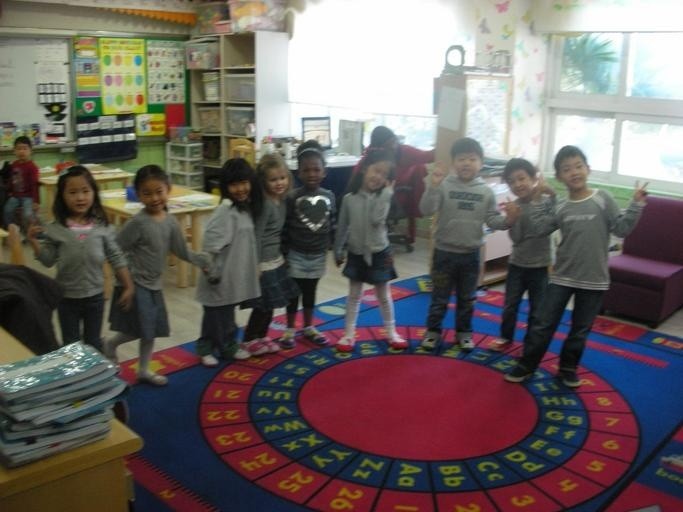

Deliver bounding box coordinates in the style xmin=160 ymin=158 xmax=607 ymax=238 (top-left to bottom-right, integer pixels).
xmin=228 ymin=136 xmax=258 ymax=170
xmin=0 ymin=222 xmax=23 ymax=269
xmin=591 ymin=192 xmax=682 ymax=329
xmin=379 ymin=169 xmax=413 ymax=252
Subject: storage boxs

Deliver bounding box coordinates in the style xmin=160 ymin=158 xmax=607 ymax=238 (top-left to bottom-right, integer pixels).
xmin=168 ymin=126 xmax=204 ymax=146
xmin=222 ymin=105 xmax=253 ymax=136
xmin=182 ymin=38 xmax=219 ymax=70
xmin=193 ymin=0 xmax=285 ymax=34
xmin=224 ymin=70 xmax=256 ymax=103
xmin=191 ymin=103 xmax=219 ymax=134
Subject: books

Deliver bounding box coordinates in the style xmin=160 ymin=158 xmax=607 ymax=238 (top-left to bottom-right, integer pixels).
xmin=1 ymin=338 xmax=128 ymax=469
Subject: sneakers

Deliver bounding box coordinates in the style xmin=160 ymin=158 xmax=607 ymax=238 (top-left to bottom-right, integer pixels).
xmin=383 ymin=331 xmax=410 ymax=354
xmin=193 ymin=323 xmax=330 ymax=368
xmin=332 ymin=334 xmax=360 ymax=354
xmin=558 ymin=369 xmax=584 ymax=388
xmin=131 ymin=368 xmax=170 ymax=388
xmin=491 ymin=336 xmax=515 ymax=353
xmin=421 ymin=331 xmax=445 ymax=353
xmin=455 ymin=333 xmax=475 ymax=353
xmin=101 ymin=335 xmax=121 ymax=366
xmin=501 ymin=365 xmax=536 ymax=385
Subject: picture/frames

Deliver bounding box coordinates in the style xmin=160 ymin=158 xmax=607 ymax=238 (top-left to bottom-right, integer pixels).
xmin=300 ymin=115 xmax=331 ymax=151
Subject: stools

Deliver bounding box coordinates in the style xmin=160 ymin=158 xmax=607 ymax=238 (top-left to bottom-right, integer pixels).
xmin=14 ymin=203 xmax=43 ymax=243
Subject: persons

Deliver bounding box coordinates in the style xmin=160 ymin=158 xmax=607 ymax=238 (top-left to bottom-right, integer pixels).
xmin=279 ymin=140 xmax=343 ymax=349
xmin=103 ymin=164 xmax=215 ymax=387
xmin=488 ymin=158 xmax=557 ymax=352
xmin=418 ymin=138 xmax=519 ymax=354
xmin=196 ymin=157 xmax=264 ymax=366
xmin=30 ymin=165 xmax=134 ymax=346
xmin=337 ymin=147 xmax=409 ymax=352
xmin=503 ymin=146 xmax=650 ymax=389
xmin=362 ymin=127 xmax=435 ymax=244
xmin=3 ymin=137 xmax=40 ymax=244
xmin=239 ymin=155 xmax=303 ymax=356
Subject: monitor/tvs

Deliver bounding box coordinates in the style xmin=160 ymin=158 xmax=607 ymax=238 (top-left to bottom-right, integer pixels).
xmin=302 ymin=117 xmax=331 ymax=149
xmin=339 ymin=120 xmax=364 ymax=156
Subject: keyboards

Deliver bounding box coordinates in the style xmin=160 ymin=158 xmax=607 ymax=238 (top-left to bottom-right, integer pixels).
xmin=322 ymin=156 xmax=363 ymax=165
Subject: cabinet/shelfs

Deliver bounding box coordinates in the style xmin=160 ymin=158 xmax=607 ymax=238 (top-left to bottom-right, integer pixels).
xmin=432 ymin=178 xmax=524 ymax=287
xmin=434 ymin=74 xmax=513 ymax=183
xmin=165 ymin=142 xmax=203 ymax=192
xmin=189 ymin=30 xmax=294 ymax=193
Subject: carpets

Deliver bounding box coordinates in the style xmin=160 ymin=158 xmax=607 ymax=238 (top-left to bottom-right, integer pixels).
xmin=601 ymin=419 xmax=682 ymax=512
xmin=100 ymin=273 xmax=682 ymax=512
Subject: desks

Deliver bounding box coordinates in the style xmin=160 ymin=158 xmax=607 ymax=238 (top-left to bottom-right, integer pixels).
xmin=92 ymin=182 xmax=226 ymax=291
xmin=33 ymin=160 xmax=136 ymax=221
xmin=252 ymin=149 xmax=367 ymax=255
xmin=0 ymin=320 xmax=147 ymax=512
